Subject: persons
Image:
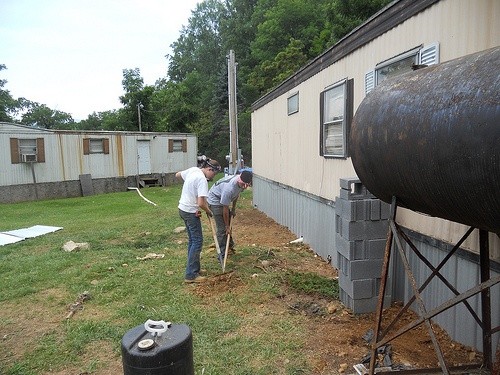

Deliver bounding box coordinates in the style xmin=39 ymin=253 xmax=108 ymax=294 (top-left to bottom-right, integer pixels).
xmin=175 ymin=159 xmax=221 ymax=283
xmin=207 ymin=171 xmax=252 ymax=255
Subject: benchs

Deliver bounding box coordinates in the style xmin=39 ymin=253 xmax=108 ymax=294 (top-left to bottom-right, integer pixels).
xmin=140 ymin=178 xmax=158 ymax=187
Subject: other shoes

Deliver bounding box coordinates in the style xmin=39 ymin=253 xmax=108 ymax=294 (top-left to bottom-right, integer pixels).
xmin=229 ymin=245 xmax=236 ymax=251
xmin=227 ymin=249 xmax=233 ymax=255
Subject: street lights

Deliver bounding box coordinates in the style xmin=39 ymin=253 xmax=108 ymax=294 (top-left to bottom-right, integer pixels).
xmin=138 ymin=102 xmax=143 ymax=132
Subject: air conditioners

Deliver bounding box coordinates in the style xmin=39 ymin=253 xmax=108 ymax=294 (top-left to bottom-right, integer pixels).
xmin=20 ymin=154 xmax=37 ymax=163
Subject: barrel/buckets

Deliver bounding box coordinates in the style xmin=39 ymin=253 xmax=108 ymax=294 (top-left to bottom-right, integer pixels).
xmin=122 ymin=320 xmax=194 ymax=375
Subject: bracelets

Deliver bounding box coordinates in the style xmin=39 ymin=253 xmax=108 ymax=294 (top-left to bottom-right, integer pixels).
xmin=232 ymin=208 xmax=235 ymax=211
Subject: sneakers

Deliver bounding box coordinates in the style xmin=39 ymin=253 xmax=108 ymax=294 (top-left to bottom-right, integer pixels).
xmin=185 ymin=276 xmax=206 ymax=282
xmin=198 ymin=269 xmax=207 ymax=274
